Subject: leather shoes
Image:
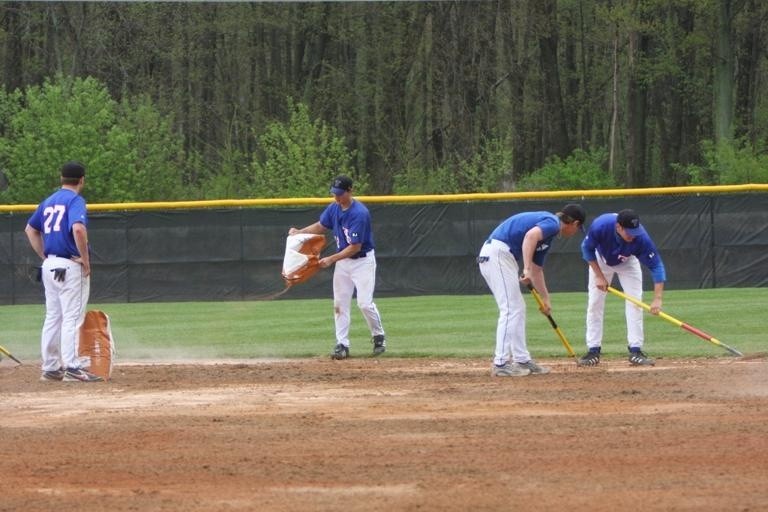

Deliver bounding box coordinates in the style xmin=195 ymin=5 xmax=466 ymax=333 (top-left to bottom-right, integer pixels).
xmin=476 ymin=255 xmax=490 ymax=266
xmin=52 ymin=268 xmax=69 ymax=283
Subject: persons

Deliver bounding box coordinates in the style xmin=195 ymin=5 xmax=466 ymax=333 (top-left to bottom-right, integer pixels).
xmin=24 ymin=161 xmax=103 ymax=382
xmin=477 ymin=203 xmax=586 ymax=376
xmin=288 ymin=176 xmax=386 ymax=360
xmin=580 ymin=209 xmax=667 ymax=366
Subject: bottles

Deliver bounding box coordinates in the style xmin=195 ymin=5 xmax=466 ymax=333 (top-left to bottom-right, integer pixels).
xmin=330 ymin=175 xmax=355 ymax=195
xmin=562 ymin=202 xmax=588 ymax=234
xmin=60 ymin=160 xmax=86 ymax=179
xmin=615 ymin=207 xmax=646 ymax=237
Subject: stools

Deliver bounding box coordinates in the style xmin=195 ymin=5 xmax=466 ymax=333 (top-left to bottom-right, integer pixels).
xmin=484 ymin=238 xmax=519 ymax=260
xmin=348 ymin=250 xmax=371 ymax=259
xmin=43 ymin=252 xmax=66 ymax=258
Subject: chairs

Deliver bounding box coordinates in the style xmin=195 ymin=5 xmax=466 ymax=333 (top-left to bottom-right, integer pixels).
xmin=576 ymin=352 xmax=604 ymax=367
xmin=330 ymin=344 xmax=350 ymax=360
xmin=63 ymin=368 xmax=105 ymax=383
xmin=39 ymin=370 xmax=64 ymax=382
xmin=628 ymin=352 xmax=656 ymax=367
xmin=373 ymin=335 xmax=387 ymax=354
xmin=491 ymin=361 xmax=552 ymax=378
